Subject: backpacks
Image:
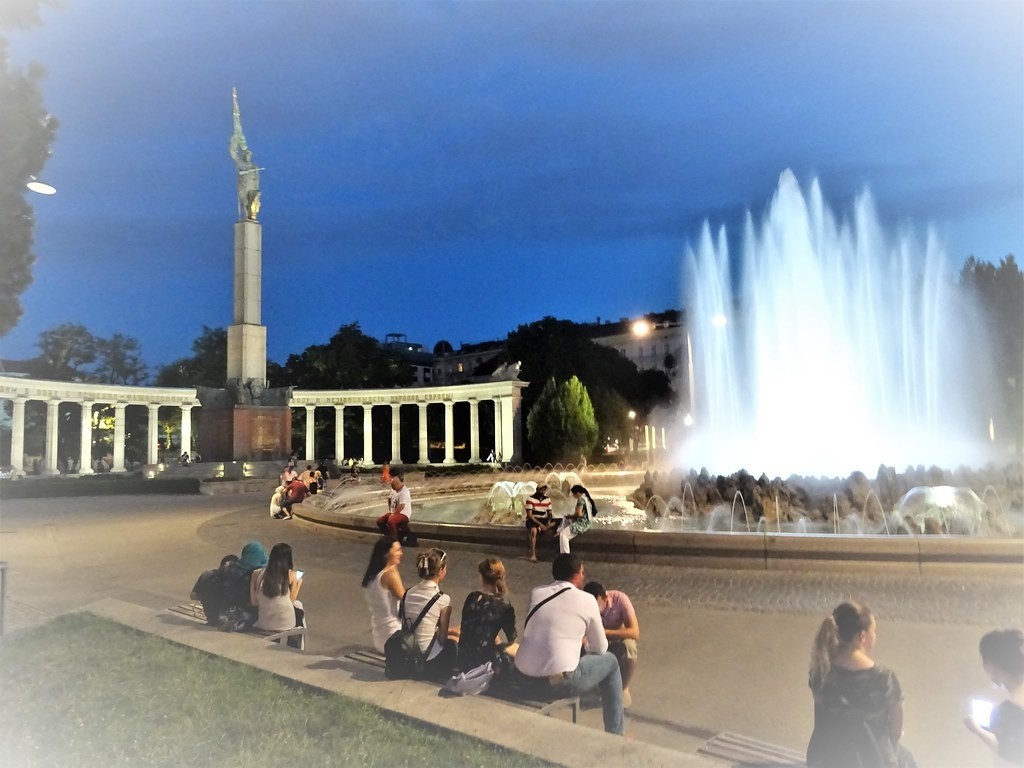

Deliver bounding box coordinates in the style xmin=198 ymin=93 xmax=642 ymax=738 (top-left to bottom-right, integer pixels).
xmin=384 ymin=586 xmax=443 ymax=676
xmin=807 ymin=664 xmax=899 ymax=768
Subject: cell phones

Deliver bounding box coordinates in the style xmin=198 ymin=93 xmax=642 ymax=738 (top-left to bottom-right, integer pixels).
xmin=971 ymin=699 xmax=992 ymax=727
xmin=296 ymin=570 xmax=304 ymax=580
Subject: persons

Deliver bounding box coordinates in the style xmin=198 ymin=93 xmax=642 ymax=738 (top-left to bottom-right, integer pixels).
xmin=190 ymin=542 xmax=306 ymax=651
xmin=525 ymin=483 xmax=563 ymax=562
xmin=182 ymin=452 xmax=190 ymax=463
xmin=458 ymin=556 xmax=520 ymax=685
xmin=583 ymin=581 xmax=640 ymax=708
xmin=270 ymin=446 xmax=363 ymax=520
xmin=399 ymin=548 xmax=461 ymax=679
xmin=377 ymin=462 xmax=412 ymax=540
xmin=486 ymin=449 xmax=502 ymax=463
xmin=512 ymin=553 xmax=625 ymax=736
xmin=961 ymin=628 xmax=1024 ymax=767
xmin=361 ymin=537 xmax=405 ymax=655
xmin=806 ymin=601 xmax=918 ymax=768
xmin=554 ymin=485 xmax=593 ymax=553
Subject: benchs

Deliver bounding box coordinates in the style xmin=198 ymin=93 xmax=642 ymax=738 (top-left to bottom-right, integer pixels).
xmin=690 ymin=731 xmax=810 ymax=768
xmin=337 ymin=645 xmax=580 ymax=724
xmin=163 ymin=602 xmax=309 ymax=654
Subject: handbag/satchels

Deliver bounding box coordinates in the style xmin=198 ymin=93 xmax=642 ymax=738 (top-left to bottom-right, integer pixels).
xmin=446 ymin=661 xmax=494 ymax=696
xmin=217 ymin=606 xmax=248 ymax=632
xmin=398 ymin=532 xmax=417 ymax=547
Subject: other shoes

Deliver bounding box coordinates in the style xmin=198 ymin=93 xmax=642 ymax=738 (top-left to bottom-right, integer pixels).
xmin=283 ymin=516 xmax=291 ymax=520
xmin=529 ymin=549 xmax=537 ymax=563
xmin=623 ymin=685 xmax=632 ymax=709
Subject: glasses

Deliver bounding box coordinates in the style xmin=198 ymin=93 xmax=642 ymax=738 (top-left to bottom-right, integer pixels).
xmin=432 ymin=548 xmax=446 ymax=561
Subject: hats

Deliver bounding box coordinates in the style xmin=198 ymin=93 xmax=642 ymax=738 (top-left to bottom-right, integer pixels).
xmin=537 ymin=483 xmax=547 ymax=489
xmin=237 ymin=541 xmax=267 ymax=567
